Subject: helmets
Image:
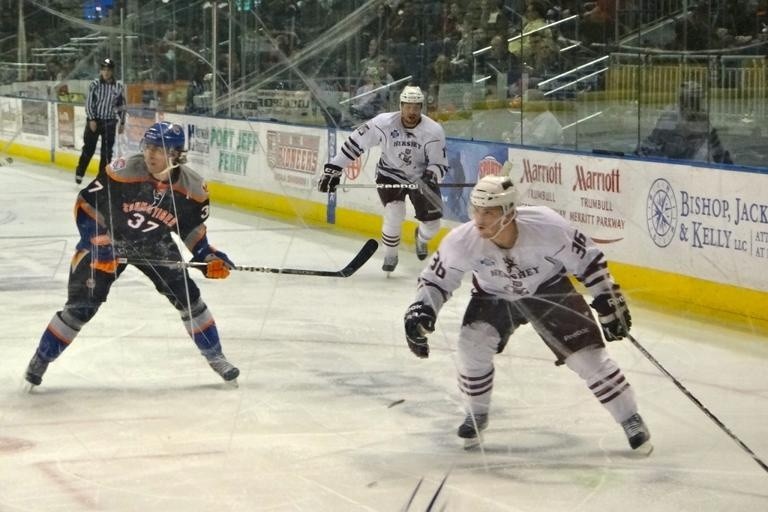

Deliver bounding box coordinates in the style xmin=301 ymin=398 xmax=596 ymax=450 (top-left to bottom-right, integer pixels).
xmin=677 ymin=81 xmax=709 ymax=100
xmin=399 ymin=85 xmax=425 ymax=107
xmin=470 ymin=174 xmax=523 ymax=208
xmin=101 ymin=57 xmax=114 ymax=70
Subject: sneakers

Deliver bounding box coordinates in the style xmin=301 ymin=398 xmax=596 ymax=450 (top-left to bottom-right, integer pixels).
xmin=208 ymin=354 xmax=240 ymax=382
xmin=383 ymin=255 xmax=399 ymax=272
xmin=414 ymin=227 xmax=428 ymax=263
xmin=458 ymin=414 xmax=489 ymax=439
xmin=25 ymin=352 xmax=50 ymax=385
xmin=75 ymin=168 xmax=82 ymax=184
xmin=620 ymin=413 xmax=651 ymax=451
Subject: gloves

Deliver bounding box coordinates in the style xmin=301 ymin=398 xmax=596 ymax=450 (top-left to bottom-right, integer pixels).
xmin=317 ymin=164 xmax=343 ymax=193
xmin=402 ymin=300 xmax=437 ymax=359
xmin=89 ymin=229 xmax=120 ymax=275
xmin=415 ymin=169 xmax=438 ymax=197
xmin=588 ymin=283 xmax=632 ymax=343
xmin=189 ymin=245 xmax=237 ymax=279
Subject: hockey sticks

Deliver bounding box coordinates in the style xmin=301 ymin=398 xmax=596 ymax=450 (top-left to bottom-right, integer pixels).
xmin=120 ymin=239 xmax=378 ymax=277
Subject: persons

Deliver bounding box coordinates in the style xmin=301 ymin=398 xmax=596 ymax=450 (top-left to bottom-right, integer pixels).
xmin=25 ymin=122 xmax=240 ymax=385
xmin=444 ymin=90 xmax=564 ymax=145
xmin=632 ymin=81 xmax=732 ymax=164
xmin=317 ymin=86 xmax=449 ymax=273
xmin=403 ymin=178 xmax=650 ymax=450
xmin=2 ymin=2 xmax=767 ymax=132
